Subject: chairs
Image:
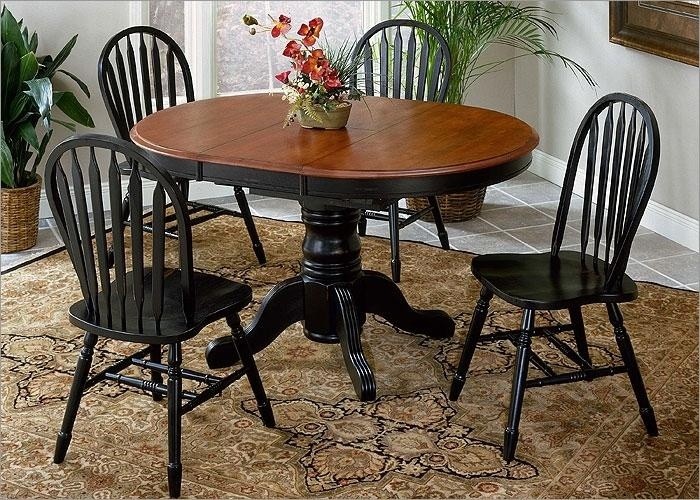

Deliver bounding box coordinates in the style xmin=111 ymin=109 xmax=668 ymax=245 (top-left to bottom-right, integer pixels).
xmin=43 ymin=133 xmax=275 ymax=498
xmin=348 ymin=20 xmax=451 ymax=284
xmin=449 ymin=92 xmax=661 ymax=461
xmin=97 ymin=25 xmax=267 ymax=272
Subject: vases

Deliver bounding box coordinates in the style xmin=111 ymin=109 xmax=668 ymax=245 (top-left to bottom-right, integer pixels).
xmin=295 ymin=102 xmax=352 ymax=130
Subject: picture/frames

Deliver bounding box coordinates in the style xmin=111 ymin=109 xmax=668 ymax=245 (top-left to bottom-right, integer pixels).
xmin=608 ymin=0 xmax=699 ymax=66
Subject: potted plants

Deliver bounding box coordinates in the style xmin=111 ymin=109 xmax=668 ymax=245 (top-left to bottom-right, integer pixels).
xmin=1 ymin=4 xmax=96 ymax=253
xmin=382 ymin=1 xmax=602 ymax=224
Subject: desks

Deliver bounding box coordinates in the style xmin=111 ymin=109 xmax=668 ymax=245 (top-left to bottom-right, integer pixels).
xmin=128 ymin=92 xmax=540 ymax=403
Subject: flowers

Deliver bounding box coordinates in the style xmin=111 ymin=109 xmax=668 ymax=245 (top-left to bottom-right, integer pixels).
xmin=241 ymin=10 xmax=396 ymax=129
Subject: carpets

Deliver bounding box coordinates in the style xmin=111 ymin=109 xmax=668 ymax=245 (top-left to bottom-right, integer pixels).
xmin=1 ymin=201 xmax=700 ymax=500
